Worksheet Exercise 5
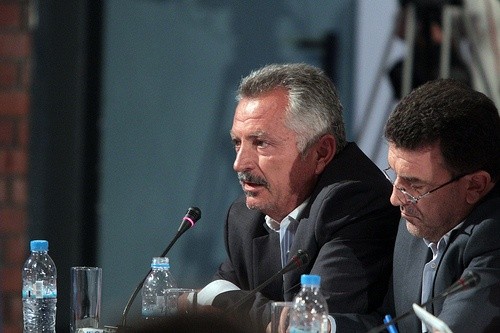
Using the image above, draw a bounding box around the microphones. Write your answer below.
[211,248,313,324]
[368,271,481,333]
[121,207,201,324]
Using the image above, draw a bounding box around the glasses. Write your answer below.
[384,166,471,204]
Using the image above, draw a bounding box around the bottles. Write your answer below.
[21,239,56,333]
[286,274,329,333]
[140,257,179,326]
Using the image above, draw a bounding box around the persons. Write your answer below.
[385,78,500,333]
[178,62,402,333]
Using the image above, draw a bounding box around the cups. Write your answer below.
[270,302,336,333]
[163,287,198,318]
[70,266,102,333]
